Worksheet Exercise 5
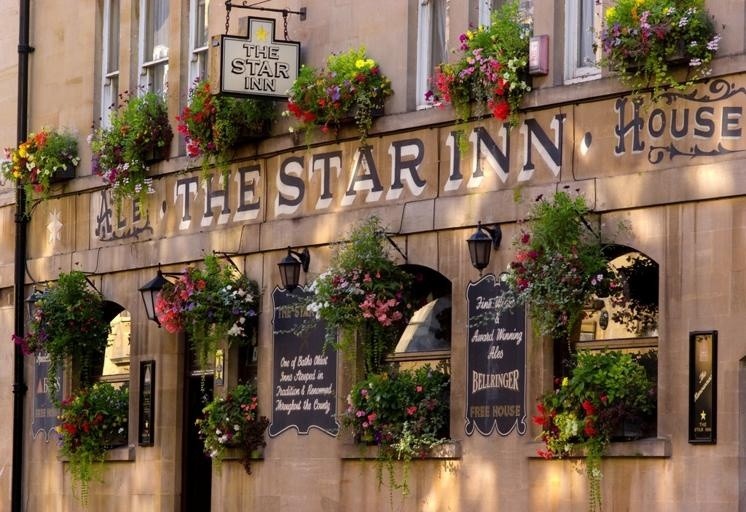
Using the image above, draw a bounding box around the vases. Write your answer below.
[469,69,532,100]
[142,140,165,161]
[613,34,695,72]
[19,158,75,185]
[236,119,270,138]
[319,97,384,127]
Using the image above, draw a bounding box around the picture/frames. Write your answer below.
[688,330,718,445]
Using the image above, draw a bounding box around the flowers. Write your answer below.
[584,0,723,114]
[175,75,282,193]
[0,124,80,221]
[281,42,394,158]
[86,82,174,218]
[425,0,534,161]
